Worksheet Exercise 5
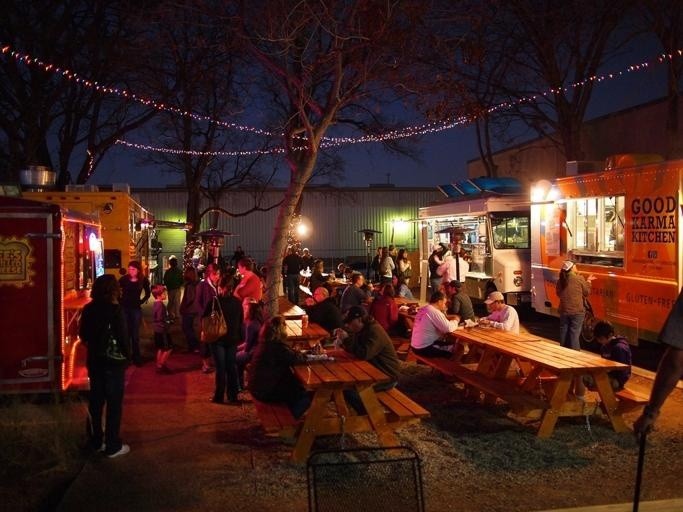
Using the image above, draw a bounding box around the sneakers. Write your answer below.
[207,395,225,404]
[154,363,176,376]
[105,444,131,459]
[200,362,217,374]
[91,438,107,454]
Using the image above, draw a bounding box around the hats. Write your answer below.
[483,290,505,305]
[560,259,575,272]
[445,279,462,288]
[340,305,368,324]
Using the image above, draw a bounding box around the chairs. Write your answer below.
[307,447,425,511]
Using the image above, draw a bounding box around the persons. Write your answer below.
[79,274,130,460]
[634,286,683,443]
[604,205,626,256]
[593,321,631,391]
[579,311,597,391]
[556,260,597,349]
[120,235,519,420]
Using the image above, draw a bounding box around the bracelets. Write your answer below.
[643,405,659,419]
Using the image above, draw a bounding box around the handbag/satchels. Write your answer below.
[103,335,133,366]
[199,309,229,344]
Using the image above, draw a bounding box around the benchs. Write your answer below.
[590,386,651,415]
[412,352,472,375]
[254,394,297,438]
[373,387,432,427]
[454,372,517,397]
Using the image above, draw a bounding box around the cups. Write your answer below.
[302,314,308,329]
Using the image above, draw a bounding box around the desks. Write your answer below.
[278,295,306,317]
[326,277,349,300]
[502,341,630,439]
[451,326,561,382]
[282,320,329,351]
[288,345,402,465]
[393,296,419,305]
[399,310,463,323]
